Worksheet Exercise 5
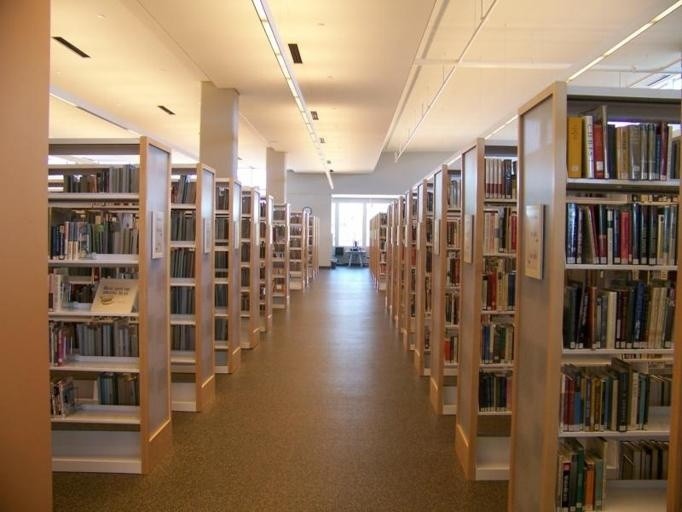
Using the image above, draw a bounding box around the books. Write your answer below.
[557,116,682,511]
[48,165,140,417]
[170,174,195,352]
[241,195,313,317]
[479,157,518,413]
[215,186,229,341]
[379,180,462,366]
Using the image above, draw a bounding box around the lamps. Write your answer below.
[251,0,334,190]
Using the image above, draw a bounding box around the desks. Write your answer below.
[345,249,367,267]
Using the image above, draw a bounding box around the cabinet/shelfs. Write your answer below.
[290,211,305,291]
[305,214,310,286]
[384,204,390,309]
[412,178,431,376]
[47,178,244,375]
[47,133,174,475]
[259,195,276,333]
[458,134,519,481]
[272,202,292,310]
[369,213,387,292]
[241,187,261,350]
[369,81,682,512]
[47,163,218,414]
[429,166,455,412]
[309,215,318,278]
[507,82,680,512]
[402,190,433,353]
[395,196,405,331]
[390,200,399,320]
